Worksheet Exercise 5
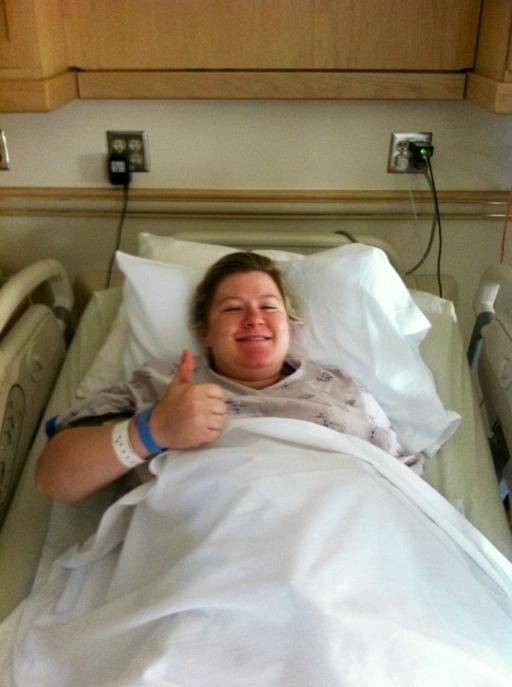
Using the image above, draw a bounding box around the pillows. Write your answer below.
[116,243,462,455]
[77,230,306,399]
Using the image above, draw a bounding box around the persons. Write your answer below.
[34,247,429,504]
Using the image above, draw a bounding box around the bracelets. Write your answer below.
[111,418,149,470]
[136,406,163,455]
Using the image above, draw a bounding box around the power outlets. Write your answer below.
[387,131,432,173]
[106,129,149,172]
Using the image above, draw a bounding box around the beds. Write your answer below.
[0,232,511,687]
